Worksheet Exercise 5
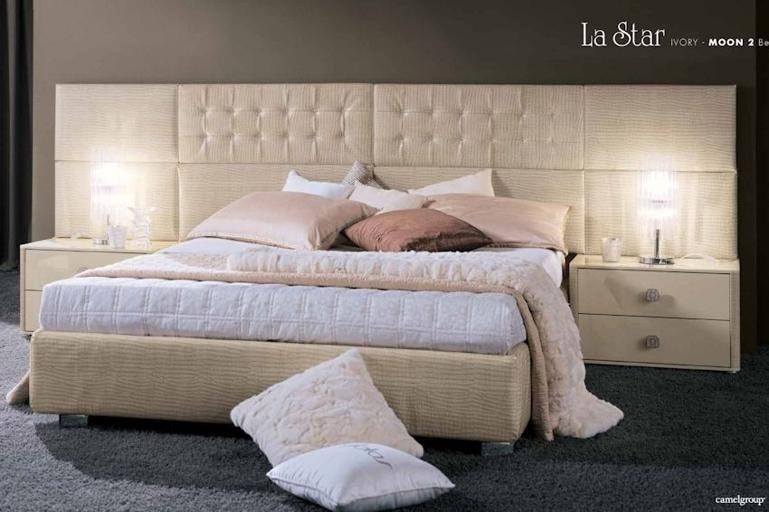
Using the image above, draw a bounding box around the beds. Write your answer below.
[10,81,738,458]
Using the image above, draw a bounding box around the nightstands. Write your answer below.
[17,232,169,336]
[565,253,740,377]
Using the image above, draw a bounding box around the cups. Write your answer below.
[107,222,125,249]
[601,236,623,261]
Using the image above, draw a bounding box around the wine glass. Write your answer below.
[125,203,157,249]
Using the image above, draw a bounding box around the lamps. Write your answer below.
[634,166,682,264]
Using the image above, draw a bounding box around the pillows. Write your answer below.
[266,438,457,512]
[227,345,422,465]
[345,181,427,211]
[342,209,491,252]
[284,169,354,199]
[408,166,498,198]
[421,194,578,252]
[176,188,379,251]
